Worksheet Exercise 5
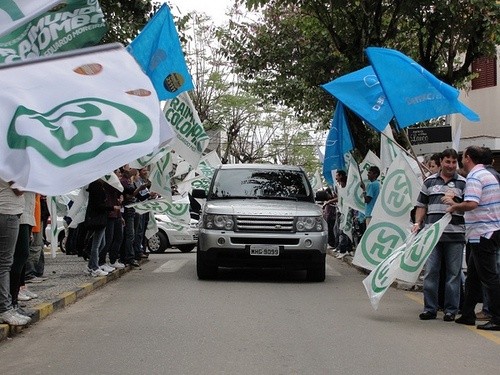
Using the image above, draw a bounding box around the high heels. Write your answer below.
[0,308,32,326]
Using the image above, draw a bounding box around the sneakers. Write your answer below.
[17,292,30,301]
[92,268,109,276]
[100,264,114,272]
[110,261,125,269]
[20,286,38,299]
[328,244,353,259]
[83,265,93,274]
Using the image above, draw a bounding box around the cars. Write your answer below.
[44,208,198,254]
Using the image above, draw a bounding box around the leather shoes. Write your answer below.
[456,317,475,325]
[477,321,500,330]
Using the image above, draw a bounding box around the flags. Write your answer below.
[0,41,177,198]
[0,0,110,65]
[45,138,226,259]
[321,99,427,273]
[388,211,452,284]
[160,89,211,170]
[364,45,482,128]
[123,2,195,102]
[317,64,395,133]
[0,0,57,31]
[362,231,418,311]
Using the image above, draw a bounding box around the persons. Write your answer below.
[0,152,183,328]
[313,141,499,332]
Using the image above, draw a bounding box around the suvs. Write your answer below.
[191,162,333,282]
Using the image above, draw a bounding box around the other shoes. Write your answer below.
[25,277,44,283]
[443,312,455,321]
[14,306,34,317]
[419,310,437,320]
[130,261,140,267]
